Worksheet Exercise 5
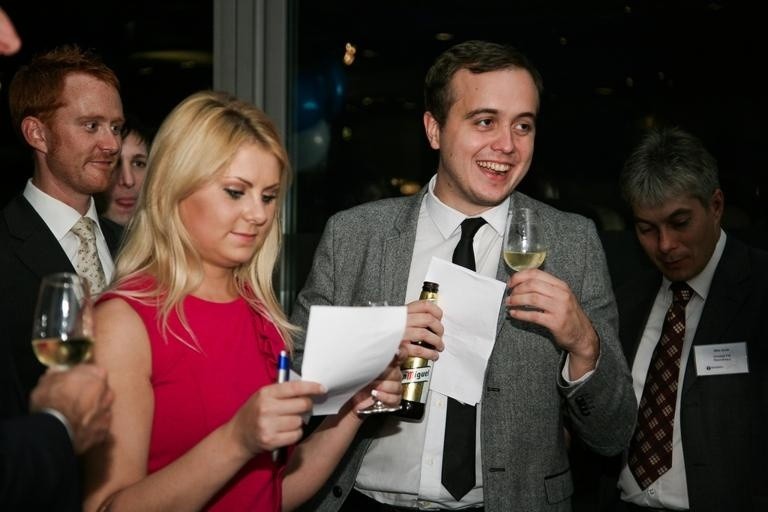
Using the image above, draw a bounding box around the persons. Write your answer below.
[100,120,152,228]
[81,92,409,512]
[572,128,768,512]
[287,39,637,511]
[0,43,126,512]
[0,7,113,512]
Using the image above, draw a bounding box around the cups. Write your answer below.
[503,208,549,274]
[31,273,95,370]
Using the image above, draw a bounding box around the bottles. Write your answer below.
[392,281,440,423]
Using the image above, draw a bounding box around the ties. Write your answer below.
[67,213,111,303]
[624,280,694,494]
[438,214,493,504]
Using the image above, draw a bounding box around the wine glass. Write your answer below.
[353,299,400,414]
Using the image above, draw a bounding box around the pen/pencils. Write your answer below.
[272,351,288,462]
